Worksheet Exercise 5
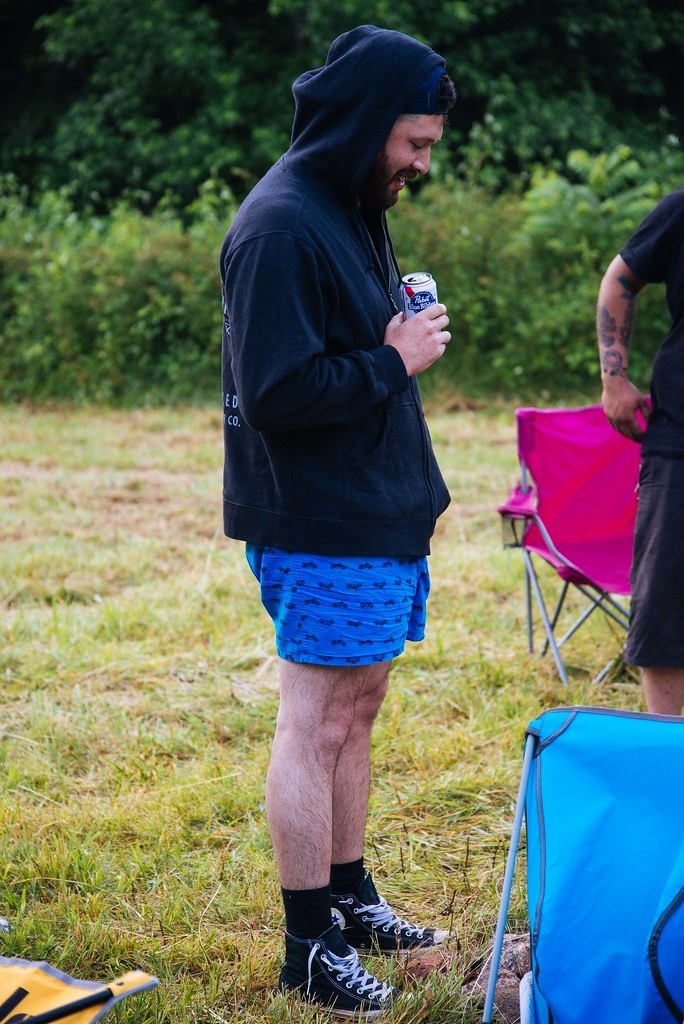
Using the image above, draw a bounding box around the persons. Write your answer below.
[222,31,465,1015]
[595,185,683,716]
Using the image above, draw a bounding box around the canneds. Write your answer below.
[399,272,438,322]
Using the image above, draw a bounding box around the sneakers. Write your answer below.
[329,873,453,955]
[277,922,427,1019]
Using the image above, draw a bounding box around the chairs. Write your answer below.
[480,705,684,1024]
[497,396,650,686]
